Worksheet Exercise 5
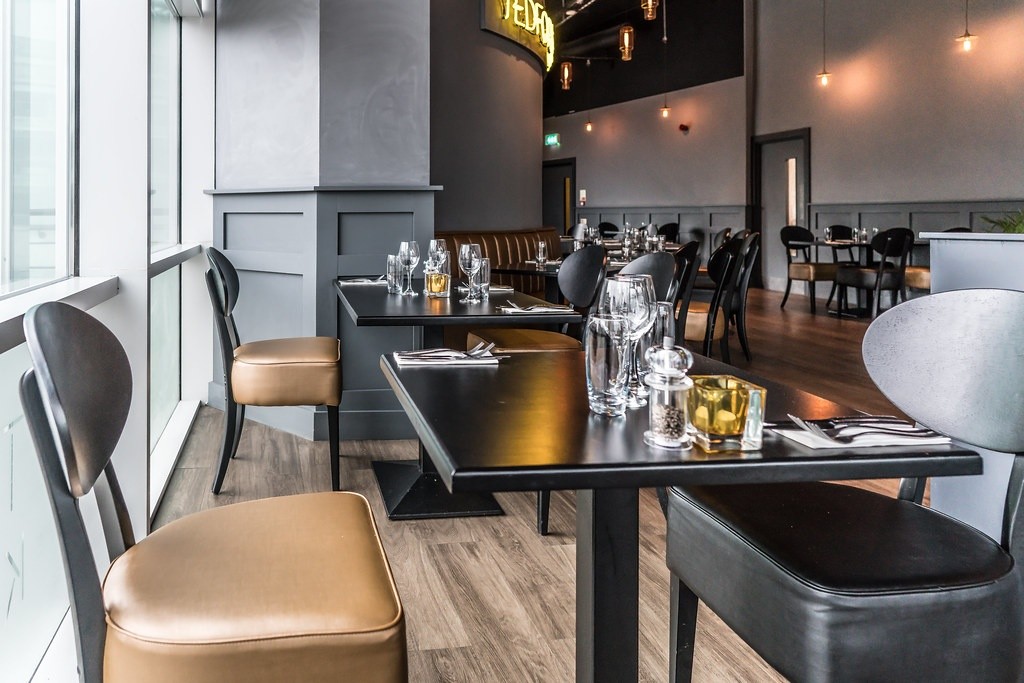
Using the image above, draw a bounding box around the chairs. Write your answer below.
[546,221,915,364]
[202,247,343,492]
[665,290,1024,683]
[17,302,409,683]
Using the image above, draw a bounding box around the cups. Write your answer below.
[425,273,450,297]
[631,302,676,396]
[472,258,489,302]
[585,313,630,417]
[852,227,879,244]
[535,241,547,268]
[574,222,668,262]
[428,239,447,264]
[386,254,403,294]
[684,375,767,454]
[824,228,832,242]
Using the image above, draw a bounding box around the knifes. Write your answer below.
[762,415,912,430]
[401,355,512,360]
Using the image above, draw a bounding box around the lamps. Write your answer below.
[640,0,659,20]
[620,22,635,61]
[560,62,572,90]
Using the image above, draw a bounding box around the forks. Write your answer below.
[395,342,496,358]
[787,414,948,445]
[507,299,569,311]
[338,274,385,282]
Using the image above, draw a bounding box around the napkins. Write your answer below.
[764,421,953,451]
[500,305,573,313]
[457,285,514,292]
[392,350,500,365]
[340,279,388,287]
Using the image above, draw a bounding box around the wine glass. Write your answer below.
[597,271,660,409]
[398,241,420,296]
[459,244,482,304]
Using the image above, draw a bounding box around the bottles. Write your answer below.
[644,335,695,450]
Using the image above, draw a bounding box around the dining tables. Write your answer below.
[380,342,982,683]
[790,235,932,318]
[487,260,631,330]
[332,269,584,520]
